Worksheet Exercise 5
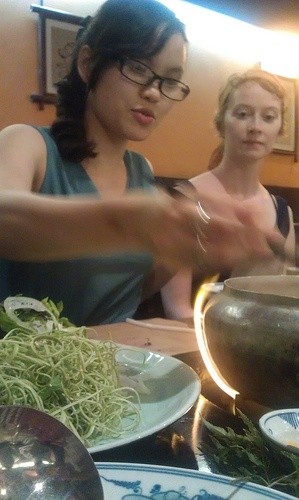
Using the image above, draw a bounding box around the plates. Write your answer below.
[0,338,201,470]
[258,408,299,453]
[93,462,299,500]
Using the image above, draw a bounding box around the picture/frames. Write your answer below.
[30,0,86,110]
[257,75,299,163]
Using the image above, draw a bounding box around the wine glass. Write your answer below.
[194,283,239,400]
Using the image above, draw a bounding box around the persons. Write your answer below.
[134,67,295,321]
[1,1,285,330]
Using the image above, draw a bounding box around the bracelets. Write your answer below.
[103,194,130,255]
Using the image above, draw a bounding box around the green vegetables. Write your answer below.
[0,295,143,447]
[197,407,299,488]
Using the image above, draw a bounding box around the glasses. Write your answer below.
[111,50,191,103]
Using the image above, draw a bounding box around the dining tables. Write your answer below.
[75,318,199,470]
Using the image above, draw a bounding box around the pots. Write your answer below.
[204,275,299,409]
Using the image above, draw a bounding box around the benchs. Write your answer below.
[133,176,299,320]
